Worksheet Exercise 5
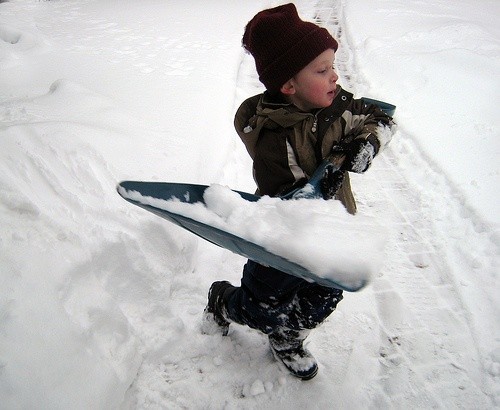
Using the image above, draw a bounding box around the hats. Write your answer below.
[240,3,338,93]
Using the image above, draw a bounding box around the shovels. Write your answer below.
[116,97,408,292]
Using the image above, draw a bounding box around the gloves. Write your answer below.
[321,169,346,201]
[331,132,380,174]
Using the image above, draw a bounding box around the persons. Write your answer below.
[201,3,397,382]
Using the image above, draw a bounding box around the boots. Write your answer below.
[269,336,319,381]
[199,281,235,337]
